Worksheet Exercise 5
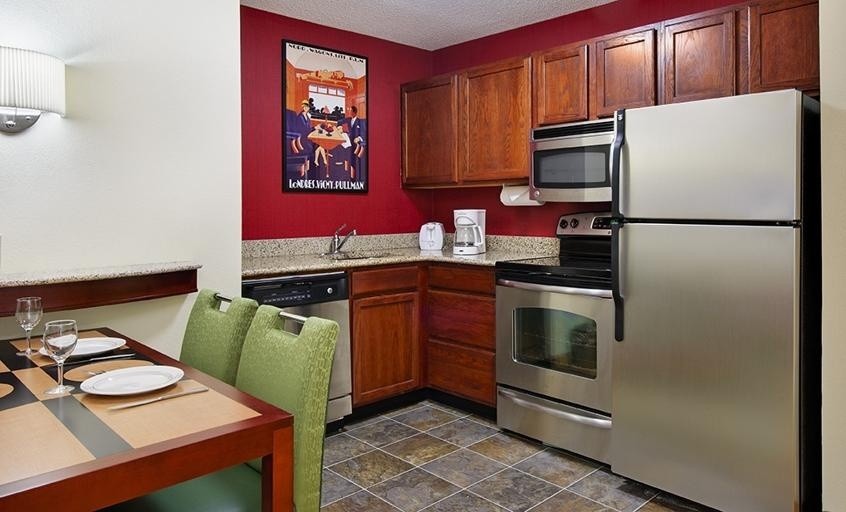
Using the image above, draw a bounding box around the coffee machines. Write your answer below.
[452,209,488,255]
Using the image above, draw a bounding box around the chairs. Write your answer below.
[179,289,260,386]
[137,306,340,511]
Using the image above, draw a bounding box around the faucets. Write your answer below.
[324,222,358,253]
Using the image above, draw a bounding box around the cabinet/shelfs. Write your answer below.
[398,56,531,190]
[534,1,819,123]
[425,262,496,420]
[350,260,421,419]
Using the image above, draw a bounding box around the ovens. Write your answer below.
[491,268,615,470]
[242,270,352,427]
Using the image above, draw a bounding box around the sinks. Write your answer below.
[327,247,397,265]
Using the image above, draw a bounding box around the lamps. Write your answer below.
[0,45,65,133]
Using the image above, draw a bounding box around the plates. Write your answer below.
[39,338,127,358]
[80,365,185,399]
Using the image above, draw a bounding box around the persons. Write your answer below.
[295,99,328,167]
[338,106,361,183]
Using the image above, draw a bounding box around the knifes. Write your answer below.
[52,354,137,369]
[108,387,210,412]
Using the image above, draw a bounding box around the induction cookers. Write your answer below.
[496,238,614,286]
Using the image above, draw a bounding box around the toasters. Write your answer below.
[418,221,445,251]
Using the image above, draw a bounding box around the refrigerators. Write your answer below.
[611,85,821,512]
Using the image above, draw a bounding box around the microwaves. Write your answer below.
[529,117,614,203]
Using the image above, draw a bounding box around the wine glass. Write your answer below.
[43,320,78,395]
[16,297,44,356]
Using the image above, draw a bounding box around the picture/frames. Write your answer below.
[281,40,370,195]
